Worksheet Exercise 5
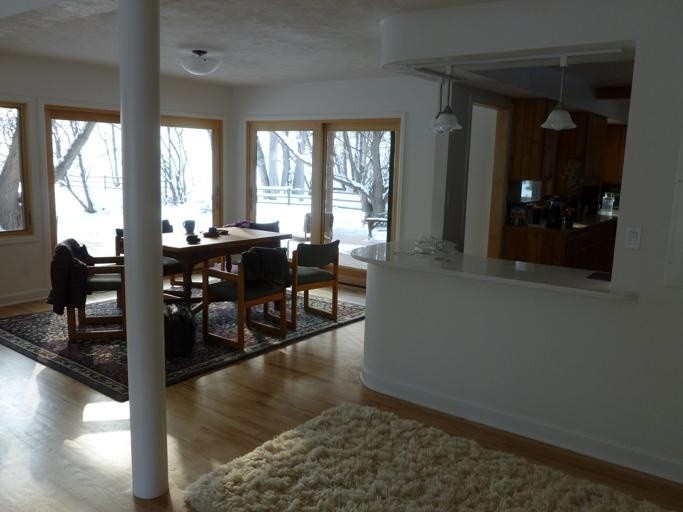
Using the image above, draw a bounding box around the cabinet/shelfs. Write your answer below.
[510,98,628,199]
[503,219,617,266]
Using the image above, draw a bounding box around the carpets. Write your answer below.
[0,278,366,403]
[184,402,669,512]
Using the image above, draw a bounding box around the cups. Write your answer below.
[184,220,195,235]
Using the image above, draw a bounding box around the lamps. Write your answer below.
[179,51,222,77]
[541,56,577,131]
[431,76,453,137]
[438,66,462,130]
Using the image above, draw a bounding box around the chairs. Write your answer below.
[162,220,186,287]
[248,221,280,249]
[56,239,127,342]
[202,248,287,350]
[116,229,124,308]
[264,240,340,329]
[287,213,334,259]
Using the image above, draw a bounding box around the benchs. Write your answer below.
[365,218,388,238]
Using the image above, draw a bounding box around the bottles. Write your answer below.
[602,192,614,216]
